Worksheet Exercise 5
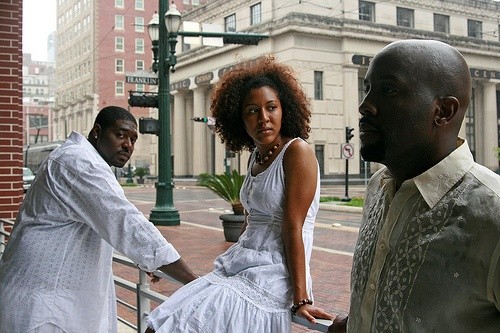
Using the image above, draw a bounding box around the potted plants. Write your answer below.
[192,168,249,242]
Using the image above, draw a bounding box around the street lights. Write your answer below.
[146,0,181,227]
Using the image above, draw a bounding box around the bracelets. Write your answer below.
[291,298,313,314]
[197,275,200,278]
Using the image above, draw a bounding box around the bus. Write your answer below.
[25,139,67,176]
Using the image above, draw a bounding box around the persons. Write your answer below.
[146,65,334,333]
[327,40,500,333]
[0,106,198,333]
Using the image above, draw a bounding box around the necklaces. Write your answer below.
[256,137,286,165]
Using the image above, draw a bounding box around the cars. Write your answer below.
[23,167,35,192]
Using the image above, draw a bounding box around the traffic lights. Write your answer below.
[191,116,208,123]
[345,126,355,143]
